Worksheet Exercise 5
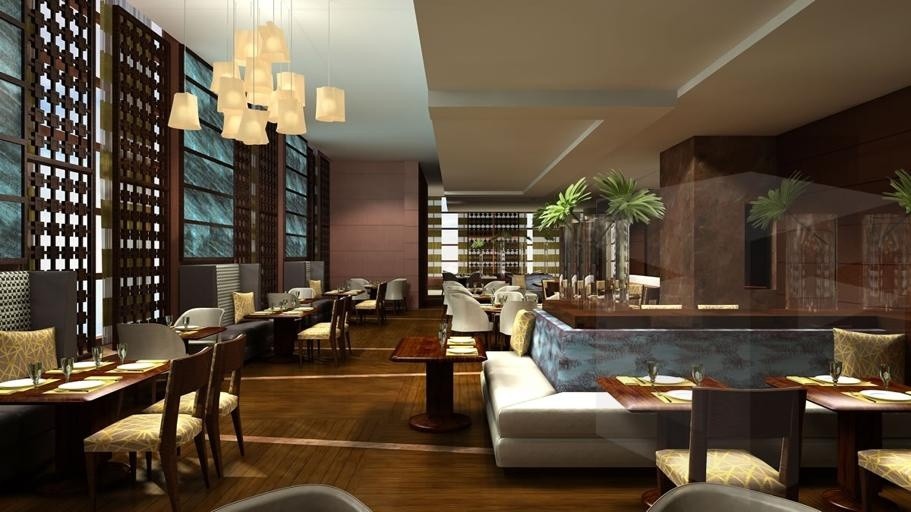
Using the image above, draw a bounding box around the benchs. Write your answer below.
[480,347,911,471]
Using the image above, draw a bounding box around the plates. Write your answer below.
[861,389,911,403]
[642,375,685,384]
[0,362,155,390]
[666,388,702,401]
[448,337,478,354]
[175,325,199,330]
[816,374,861,385]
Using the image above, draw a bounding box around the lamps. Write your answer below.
[166,0,347,146]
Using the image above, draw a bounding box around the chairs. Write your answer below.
[655,386,805,498]
[440,265,539,350]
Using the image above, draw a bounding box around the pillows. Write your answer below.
[510,310,537,356]
[831,326,908,386]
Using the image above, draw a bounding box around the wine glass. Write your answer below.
[469,261,519,268]
[644,363,662,387]
[436,321,450,352]
[90,344,102,371]
[691,363,707,389]
[116,342,127,365]
[164,314,189,333]
[268,291,301,313]
[60,356,74,380]
[879,363,891,391]
[829,361,842,390]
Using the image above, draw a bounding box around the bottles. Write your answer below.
[466,212,518,218]
[469,248,519,255]
